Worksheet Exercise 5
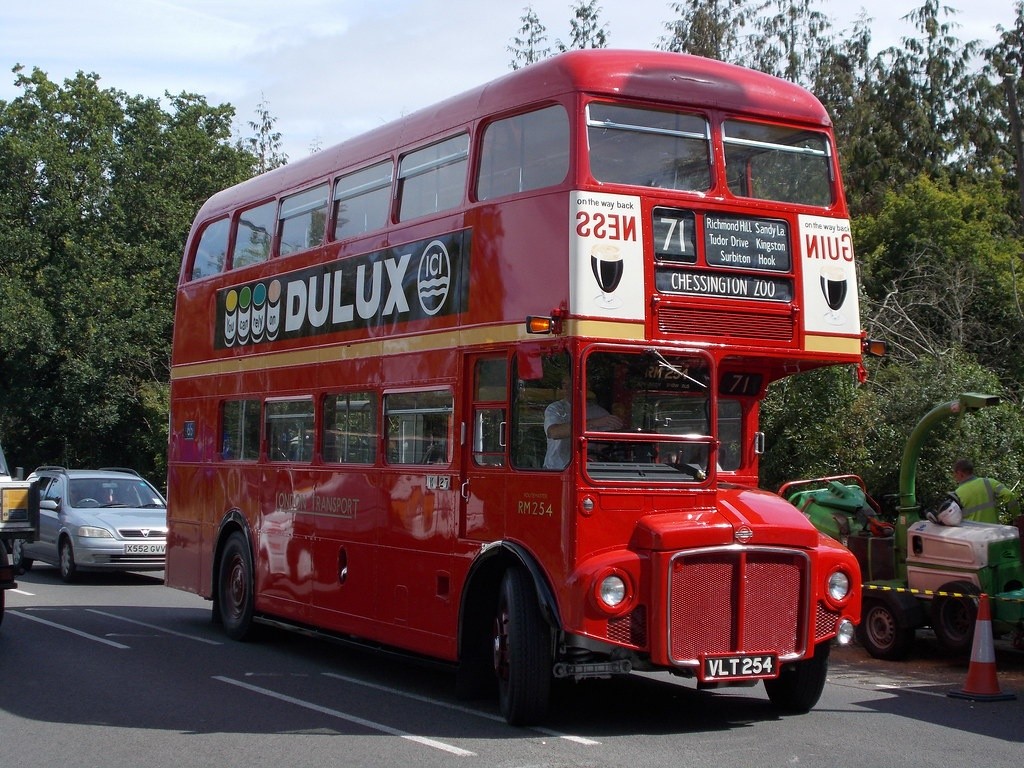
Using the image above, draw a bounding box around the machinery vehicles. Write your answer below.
[778,391,1024,665]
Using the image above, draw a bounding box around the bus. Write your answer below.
[163,49,888,731]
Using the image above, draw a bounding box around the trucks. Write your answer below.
[0,443,42,625]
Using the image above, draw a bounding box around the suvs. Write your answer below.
[9,465,170,583]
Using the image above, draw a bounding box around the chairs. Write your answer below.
[522,426,547,467]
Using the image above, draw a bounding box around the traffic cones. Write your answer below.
[946,593,1018,702]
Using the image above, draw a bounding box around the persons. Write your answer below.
[543,372,623,469]
[69,484,83,507]
[955,457,1014,524]
